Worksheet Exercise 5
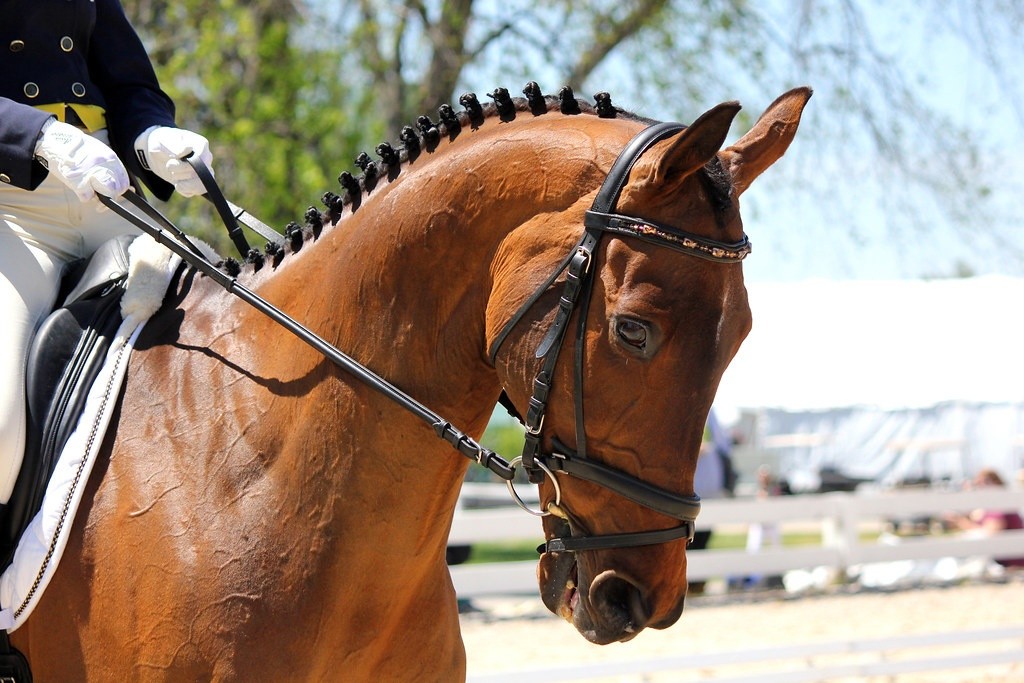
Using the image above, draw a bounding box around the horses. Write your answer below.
[0,80,813,683]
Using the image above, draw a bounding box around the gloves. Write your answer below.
[33,116,133,206]
[134,122,220,198]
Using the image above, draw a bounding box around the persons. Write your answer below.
[1,0,224,681]
[697,438,1023,592]
